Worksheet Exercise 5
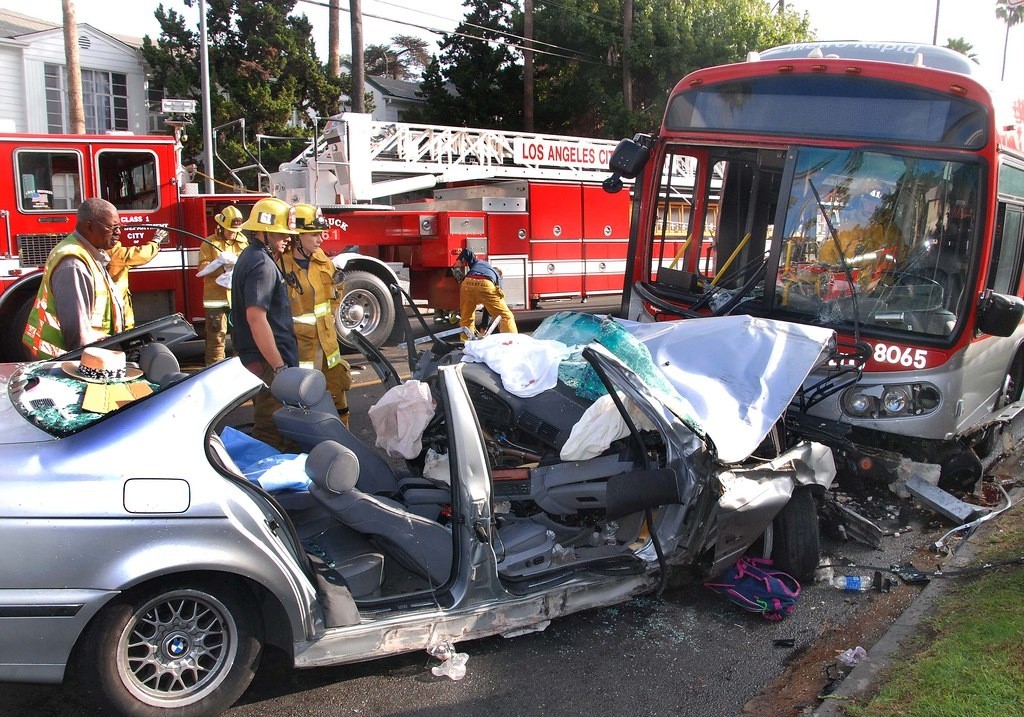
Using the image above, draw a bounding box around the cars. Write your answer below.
[0,312,839,717]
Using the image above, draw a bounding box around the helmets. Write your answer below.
[238,197,300,234]
[293,204,330,233]
[214,204,243,232]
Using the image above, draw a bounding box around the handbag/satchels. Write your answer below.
[704,552,800,623]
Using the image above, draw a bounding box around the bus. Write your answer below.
[600,37,1023,509]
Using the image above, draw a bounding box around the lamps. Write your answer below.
[161,99,196,194]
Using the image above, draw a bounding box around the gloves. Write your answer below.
[152,228,169,244]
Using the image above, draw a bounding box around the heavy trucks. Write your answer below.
[0,111,770,364]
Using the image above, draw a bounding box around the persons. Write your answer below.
[197,204,250,367]
[453,247,519,342]
[231,197,352,454]
[21,198,169,361]
[819,177,910,271]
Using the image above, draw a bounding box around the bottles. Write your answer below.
[875,312,904,323]
[829,575,872,591]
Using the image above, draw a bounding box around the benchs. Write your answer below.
[209,431,389,600]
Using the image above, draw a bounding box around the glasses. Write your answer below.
[91,220,121,233]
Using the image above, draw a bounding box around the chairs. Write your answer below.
[270,367,453,503]
[305,439,554,586]
